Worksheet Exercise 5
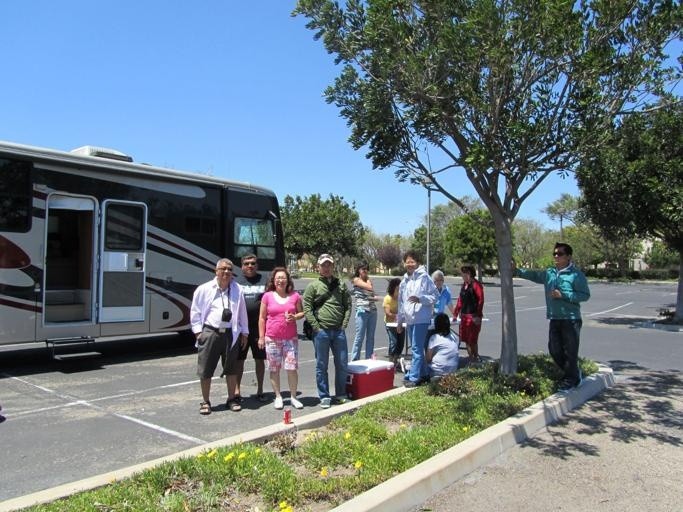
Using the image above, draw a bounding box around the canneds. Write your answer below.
[283,409,292,424]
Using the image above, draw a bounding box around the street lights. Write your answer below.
[556,206,565,243]
[419,174,435,277]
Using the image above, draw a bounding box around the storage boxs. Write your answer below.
[345,359,395,400]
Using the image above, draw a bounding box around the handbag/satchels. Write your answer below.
[304,320,311,340]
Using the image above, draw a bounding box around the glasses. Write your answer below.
[553,252,563,256]
[243,262,255,265]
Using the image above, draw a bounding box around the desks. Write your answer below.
[386,315,490,369]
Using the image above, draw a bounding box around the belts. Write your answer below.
[203,324,231,332]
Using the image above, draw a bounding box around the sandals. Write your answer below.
[200,401,210,414]
[227,397,245,411]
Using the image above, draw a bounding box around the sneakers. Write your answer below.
[319,397,352,408]
[275,398,303,409]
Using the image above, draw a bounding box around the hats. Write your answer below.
[317,253,333,264]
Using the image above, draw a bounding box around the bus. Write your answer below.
[0,141,288,363]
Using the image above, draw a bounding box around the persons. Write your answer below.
[424,313,459,380]
[510,242,590,392]
[397,250,438,388]
[257,268,305,410]
[431,268,455,317]
[347,257,380,360]
[233,251,268,405]
[302,254,351,409]
[188,257,249,415]
[452,265,484,359]
[382,279,401,372]
